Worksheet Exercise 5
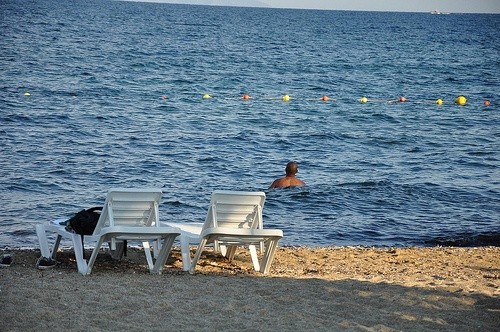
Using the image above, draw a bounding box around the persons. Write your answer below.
[269,162,305,188]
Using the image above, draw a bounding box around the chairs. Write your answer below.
[151,191,285,277]
[34,188,181,276]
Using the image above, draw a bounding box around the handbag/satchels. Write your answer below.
[60,207,104,235]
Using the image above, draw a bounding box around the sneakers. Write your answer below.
[35,257,58,270]
[0,254,12,267]
